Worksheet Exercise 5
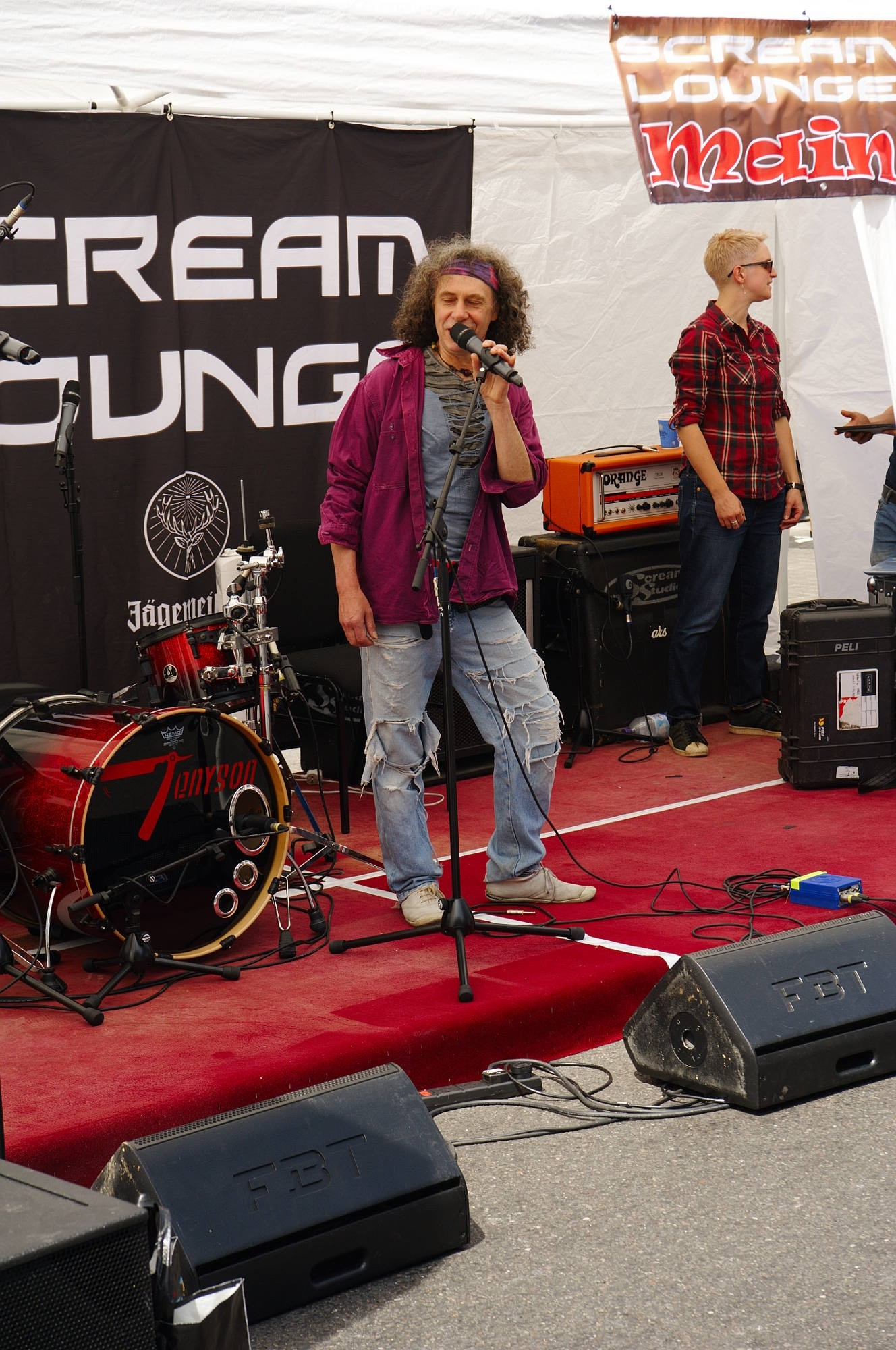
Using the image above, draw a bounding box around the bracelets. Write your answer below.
[787,483,804,492]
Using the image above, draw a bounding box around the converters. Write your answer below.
[789,872,863,910]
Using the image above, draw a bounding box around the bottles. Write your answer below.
[621,711,671,743]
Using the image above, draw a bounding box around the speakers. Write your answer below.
[619,908,896,1112]
[0,1063,470,1350]
[519,533,732,741]
[419,545,542,788]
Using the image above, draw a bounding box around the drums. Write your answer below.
[0,694,292,960]
[135,610,279,715]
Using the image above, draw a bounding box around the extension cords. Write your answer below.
[416,1072,542,1113]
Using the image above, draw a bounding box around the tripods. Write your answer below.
[542,552,667,768]
[77,352,584,1014]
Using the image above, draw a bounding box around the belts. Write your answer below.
[881,484,896,504]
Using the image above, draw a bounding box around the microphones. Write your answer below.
[53,380,81,468]
[449,322,524,388]
[0,331,42,365]
[617,575,634,629]
[241,814,290,834]
[0,192,34,243]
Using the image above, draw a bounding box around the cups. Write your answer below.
[657,418,679,449]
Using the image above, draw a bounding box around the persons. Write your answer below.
[832,406,896,567]
[318,243,596,927]
[667,228,804,757]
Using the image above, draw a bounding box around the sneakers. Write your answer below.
[668,718,708,757]
[484,859,597,904]
[729,700,782,738]
[401,881,446,927]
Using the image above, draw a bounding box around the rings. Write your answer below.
[731,521,738,525]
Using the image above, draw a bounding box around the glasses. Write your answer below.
[728,259,773,278]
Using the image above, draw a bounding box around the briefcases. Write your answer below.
[777,598,895,790]
